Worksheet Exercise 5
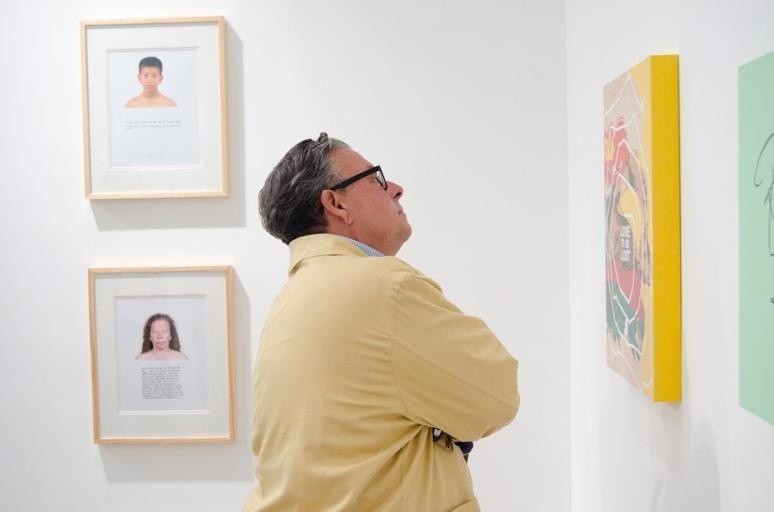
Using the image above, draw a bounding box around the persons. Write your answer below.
[122,55,178,109]
[133,313,189,362]
[241,129,522,512]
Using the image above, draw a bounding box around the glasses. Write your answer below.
[318,165,388,216]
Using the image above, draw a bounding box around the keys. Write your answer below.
[445,433,453,450]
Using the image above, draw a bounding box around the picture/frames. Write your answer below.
[79,14,232,202]
[86,264,239,447]
